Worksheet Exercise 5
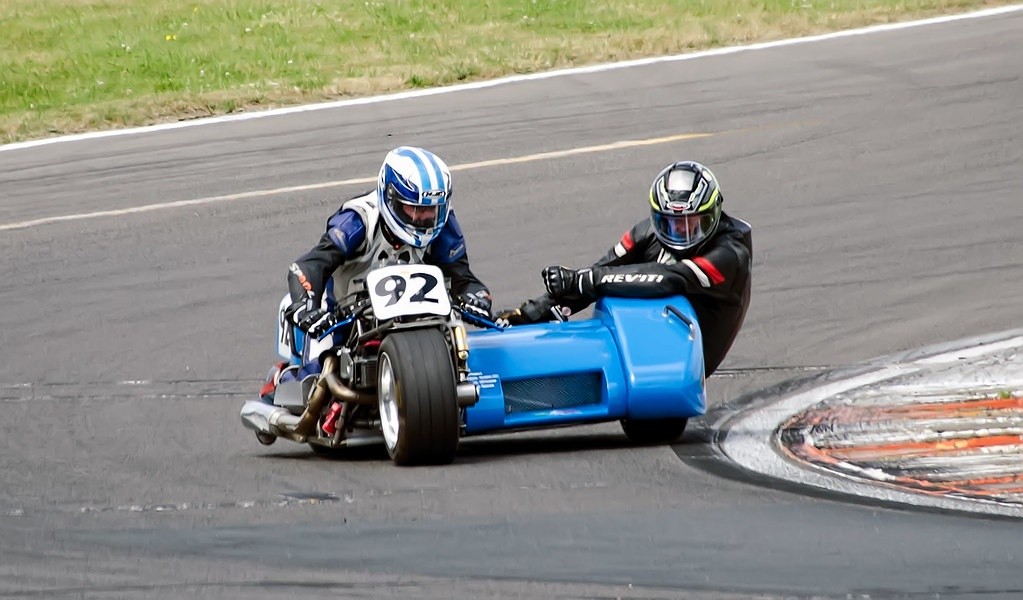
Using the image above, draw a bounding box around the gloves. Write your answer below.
[294,299,337,339]
[465,297,492,328]
[492,302,526,328]
[542,266,594,299]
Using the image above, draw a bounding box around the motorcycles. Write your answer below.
[239,257,479,467]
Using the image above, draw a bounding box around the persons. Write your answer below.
[496,161,753,379]
[255,145,492,445]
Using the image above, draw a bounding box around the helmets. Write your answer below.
[377,146,452,247]
[649,161,724,258]
